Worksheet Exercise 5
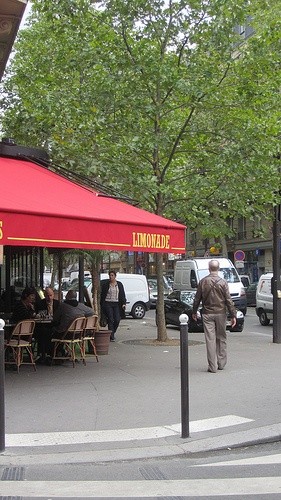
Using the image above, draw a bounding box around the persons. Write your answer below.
[100,271,126,342]
[9,287,96,366]
[191,260,236,372]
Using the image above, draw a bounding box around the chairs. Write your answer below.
[4,321,36,373]
[50,315,99,368]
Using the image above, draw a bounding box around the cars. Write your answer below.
[156,290,245,333]
[233,275,251,291]
[147,278,173,307]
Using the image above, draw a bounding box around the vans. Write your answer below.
[255,273,274,327]
[62,273,150,320]
[173,258,248,316]
[71,271,92,281]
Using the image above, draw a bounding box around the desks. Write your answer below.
[36,321,51,323]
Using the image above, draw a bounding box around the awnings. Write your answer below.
[0,157,186,254]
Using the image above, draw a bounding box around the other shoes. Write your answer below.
[110,336,115,341]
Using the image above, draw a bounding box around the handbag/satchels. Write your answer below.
[100,314,108,326]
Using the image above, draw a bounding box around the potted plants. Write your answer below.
[63,249,115,355]
